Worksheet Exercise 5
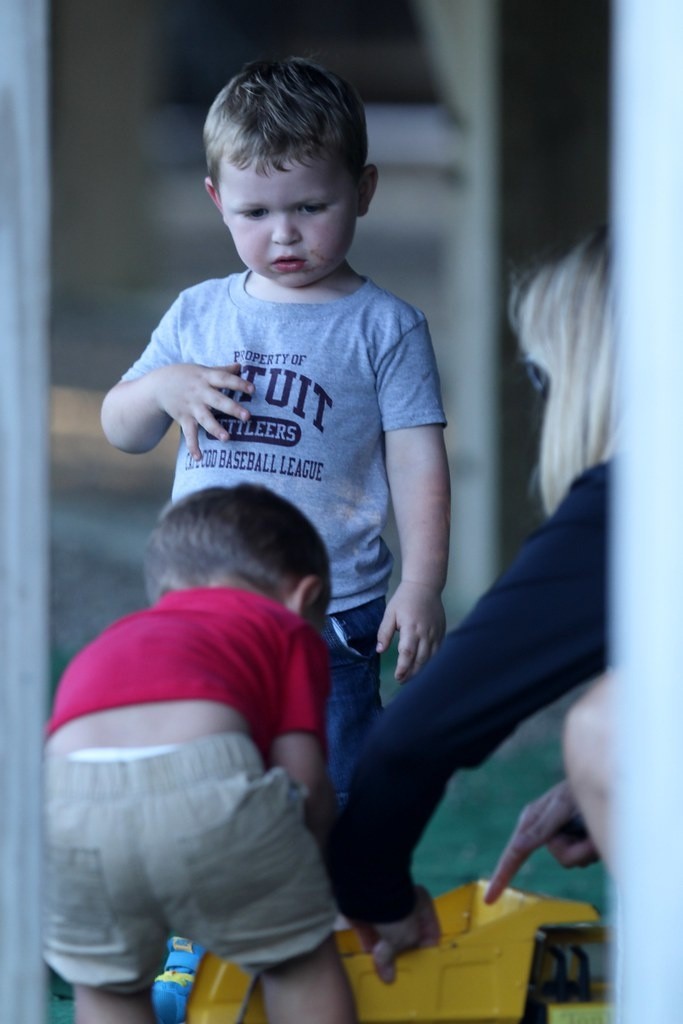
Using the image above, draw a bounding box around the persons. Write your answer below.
[98,59,450,1024]
[40,485,360,1024]
[332,229,621,985]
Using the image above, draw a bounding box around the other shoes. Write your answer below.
[150,935,206,1022]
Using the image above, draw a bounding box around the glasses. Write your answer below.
[525,356,550,399]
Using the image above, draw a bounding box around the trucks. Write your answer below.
[151,868,617,1024]
